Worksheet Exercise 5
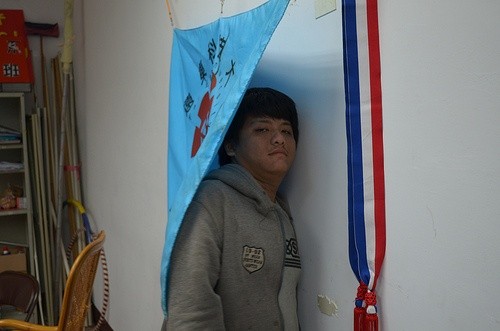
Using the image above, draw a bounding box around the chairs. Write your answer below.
[1,270,40,324]
[0,230,105,330]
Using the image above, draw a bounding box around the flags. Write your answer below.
[159,0,298,322]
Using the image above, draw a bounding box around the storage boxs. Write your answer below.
[1,8,34,91]
[0,248,28,275]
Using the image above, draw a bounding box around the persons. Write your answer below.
[161,85,305,330]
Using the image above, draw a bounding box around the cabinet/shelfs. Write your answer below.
[0,92,43,324]
